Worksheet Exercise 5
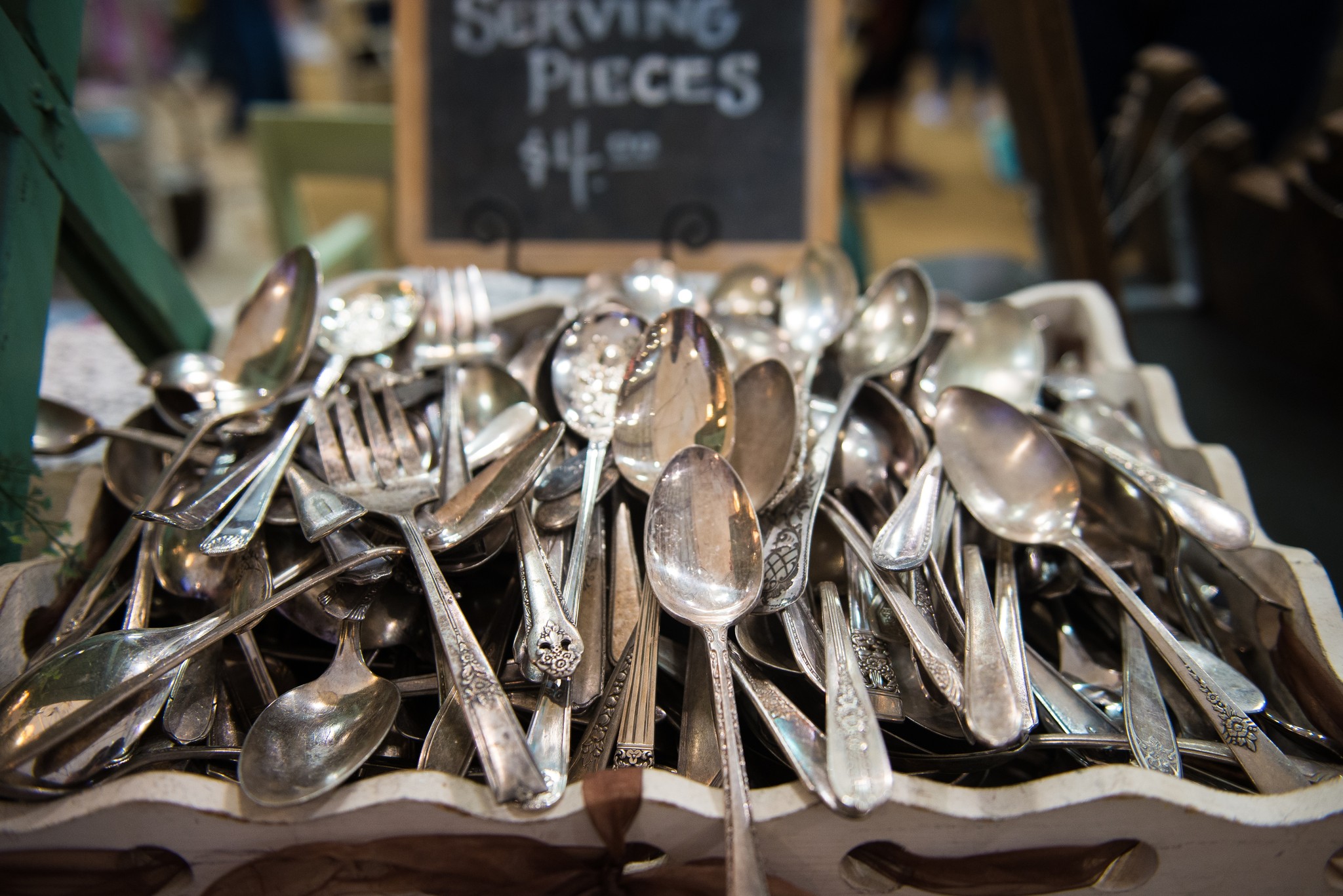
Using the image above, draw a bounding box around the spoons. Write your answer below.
[1,238,1342,896]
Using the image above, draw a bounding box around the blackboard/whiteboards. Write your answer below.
[386,0,846,285]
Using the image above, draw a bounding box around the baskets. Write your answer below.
[3,265,1343,896]
